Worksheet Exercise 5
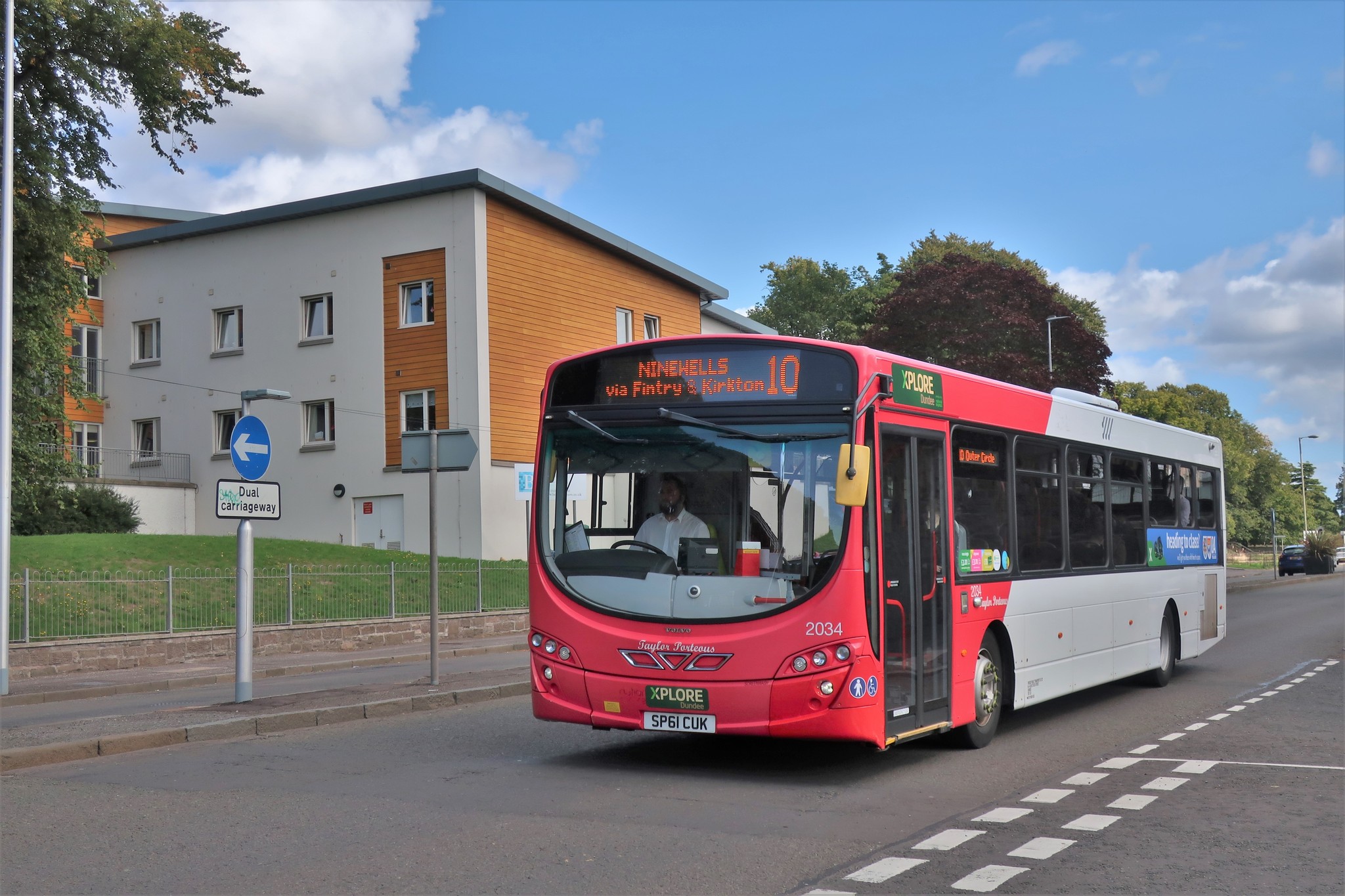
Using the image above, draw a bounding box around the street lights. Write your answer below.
[237,388,293,703]
[1299,435,1319,533]
[1046,315,1069,388]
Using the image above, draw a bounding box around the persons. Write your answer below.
[627,476,710,576]
[1179,475,1191,526]
[920,491,958,577]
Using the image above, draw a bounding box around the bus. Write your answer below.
[529,335,1226,754]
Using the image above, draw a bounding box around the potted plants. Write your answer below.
[1302,530,1341,575]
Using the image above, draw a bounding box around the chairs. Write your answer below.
[973,499,1215,573]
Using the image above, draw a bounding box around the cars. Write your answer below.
[1278,545,1306,576]
[1336,547,1345,565]
